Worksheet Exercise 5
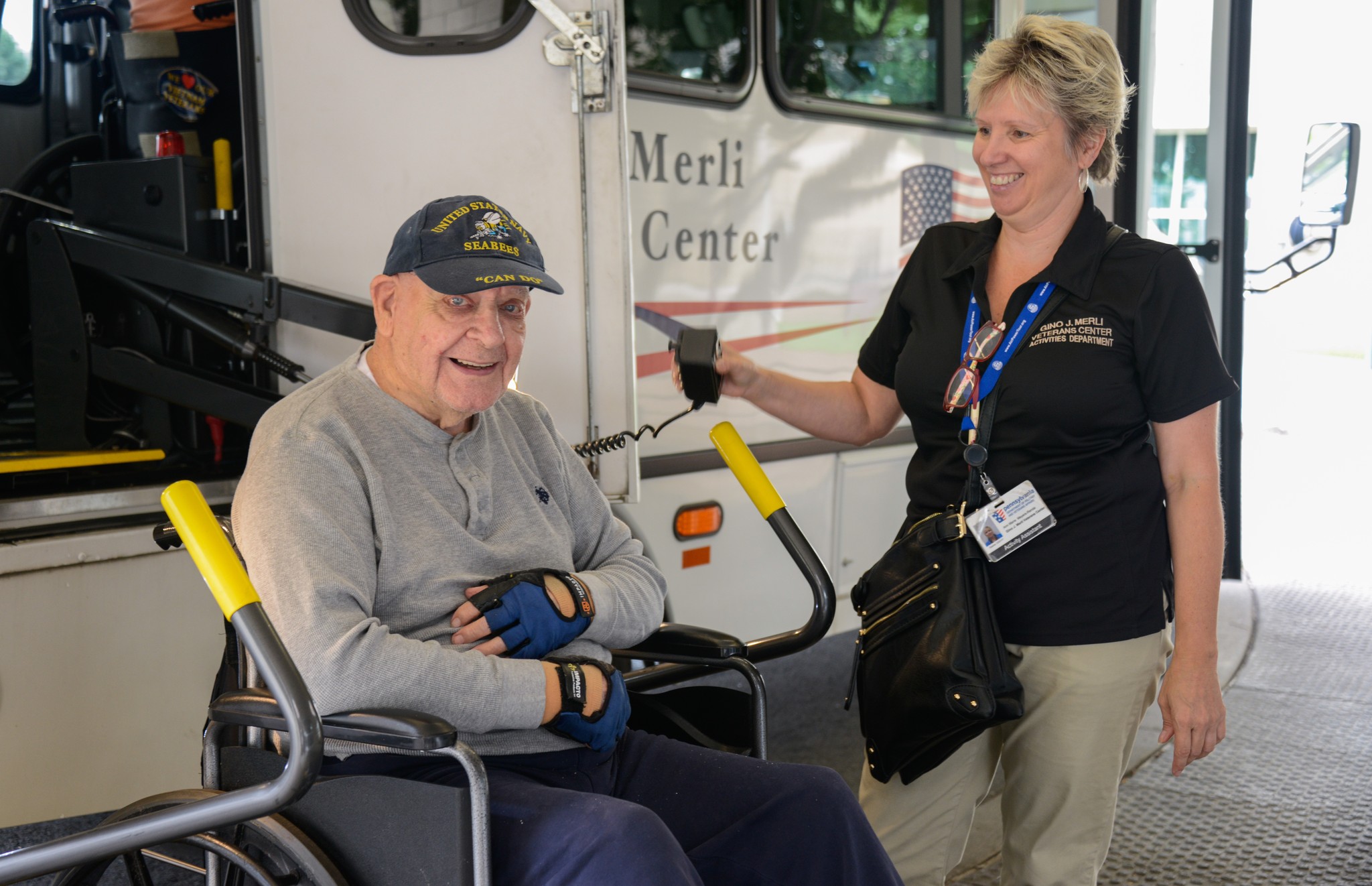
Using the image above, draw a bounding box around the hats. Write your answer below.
[382,195,566,299]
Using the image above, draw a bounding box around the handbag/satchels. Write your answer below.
[844,503,1025,787]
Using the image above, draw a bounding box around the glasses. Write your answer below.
[941,320,1007,413]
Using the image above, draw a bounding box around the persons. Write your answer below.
[669,14,1228,885]
[230,194,905,886]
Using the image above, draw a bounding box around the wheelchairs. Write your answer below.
[48,516,769,886]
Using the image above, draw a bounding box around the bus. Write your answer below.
[1,2,1360,854]
